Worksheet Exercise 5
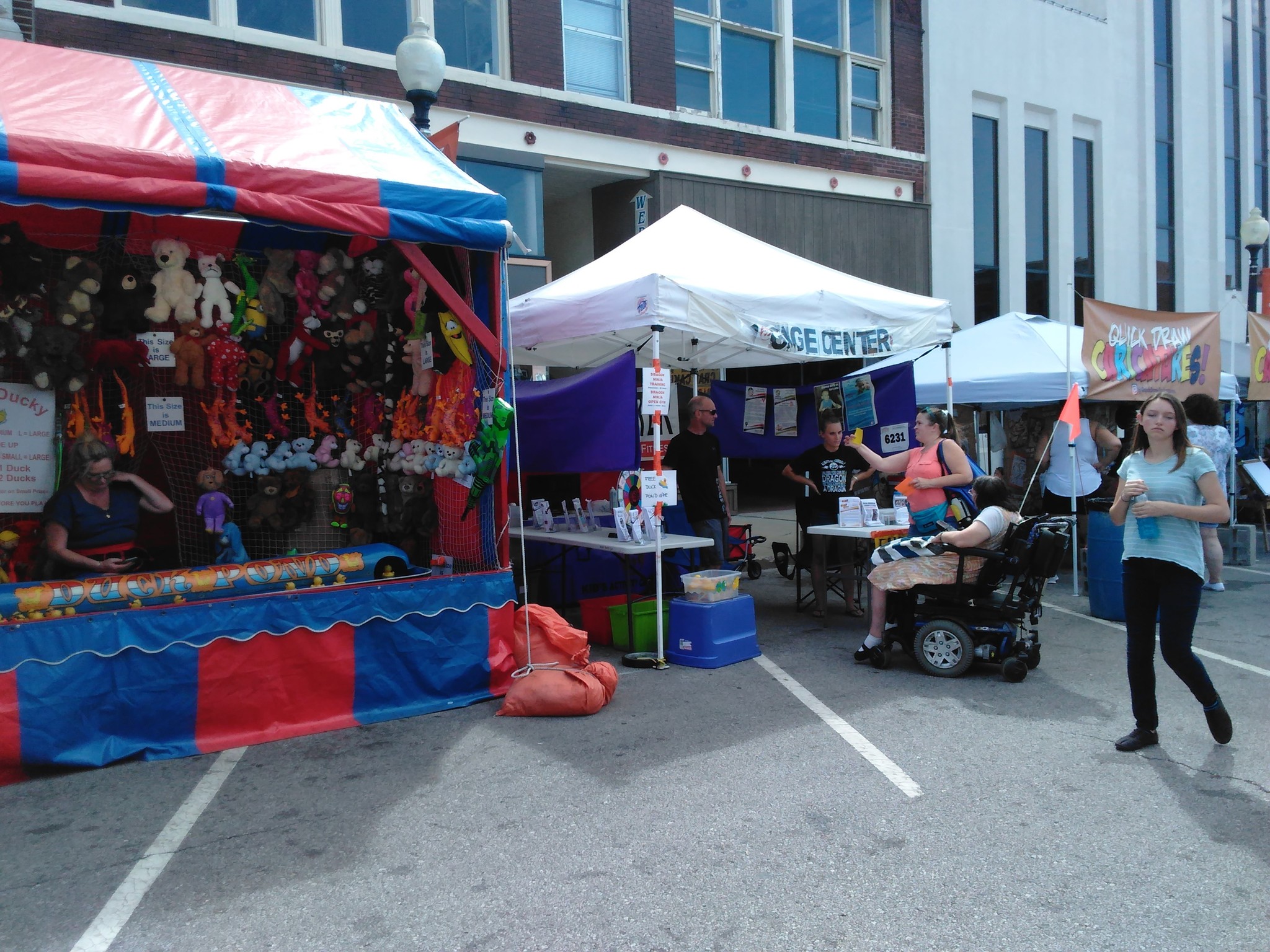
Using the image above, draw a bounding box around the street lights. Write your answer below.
[1240,206,1270,344]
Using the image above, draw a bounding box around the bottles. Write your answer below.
[1132,491,1159,540]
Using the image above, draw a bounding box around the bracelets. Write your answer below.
[939,530,948,544]
[1120,490,1132,502]
[97,559,103,573]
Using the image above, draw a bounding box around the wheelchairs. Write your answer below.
[867,510,1076,681]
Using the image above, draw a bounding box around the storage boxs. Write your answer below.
[680,569,742,603]
[579,594,656,645]
[665,594,761,668]
[606,600,671,654]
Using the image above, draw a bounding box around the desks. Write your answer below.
[508,525,714,670]
[807,523,910,631]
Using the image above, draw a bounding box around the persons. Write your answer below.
[818,390,841,415]
[842,405,976,545]
[855,378,870,396]
[1178,393,1236,591]
[1108,392,1236,750]
[781,411,879,618]
[41,433,174,583]
[849,475,1027,660]
[660,396,732,571]
[1034,406,1121,584]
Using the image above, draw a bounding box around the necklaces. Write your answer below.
[83,484,114,518]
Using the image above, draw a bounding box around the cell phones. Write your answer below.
[115,557,138,568]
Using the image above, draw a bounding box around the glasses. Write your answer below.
[86,468,116,481]
[968,489,978,496]
[694,409,717,415]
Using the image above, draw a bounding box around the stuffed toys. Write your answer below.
[0,218,500,582]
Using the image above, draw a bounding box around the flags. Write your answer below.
[1057,383,1082,440]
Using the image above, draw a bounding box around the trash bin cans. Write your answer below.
[1086,496,1165,622]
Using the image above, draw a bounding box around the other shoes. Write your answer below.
[854,643,893,661]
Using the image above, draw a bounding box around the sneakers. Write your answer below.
[1114,727,1159,750]
[1204,694,1234,744]
[1047,574,1059,584]
[1202,579,1225,592]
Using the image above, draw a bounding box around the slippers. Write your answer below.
[812,609,824,617]
[846,605,865,618]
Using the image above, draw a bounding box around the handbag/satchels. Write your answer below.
[938,439,988,518]
[906,501,949,533]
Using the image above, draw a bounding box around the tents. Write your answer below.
[507,202,958,672]
[911,310,1245,599]
